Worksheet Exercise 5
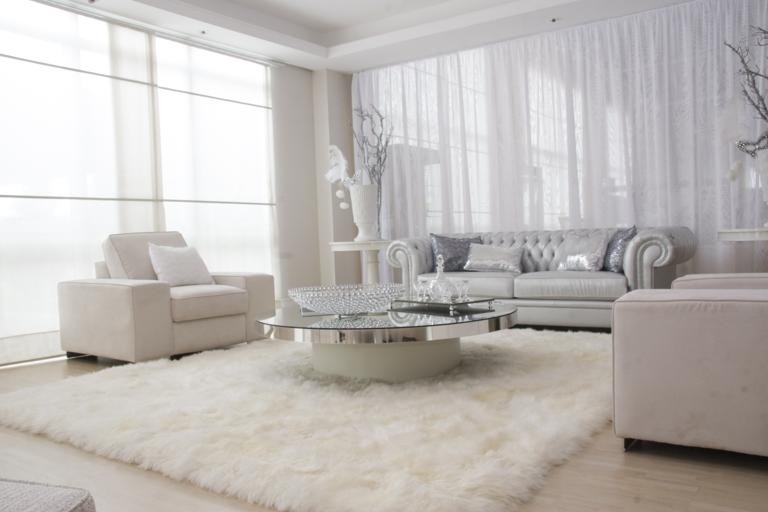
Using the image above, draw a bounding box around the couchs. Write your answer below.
[59,231,275,363]
[387,227,698,326]
[614,273,768,451]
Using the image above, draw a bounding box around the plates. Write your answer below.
[288,284,407,314]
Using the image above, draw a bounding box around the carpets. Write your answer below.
[0,326,612,511]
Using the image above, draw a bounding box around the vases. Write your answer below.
[349,183,380,243]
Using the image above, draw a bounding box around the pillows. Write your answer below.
[463,243,525,272]
[147,242,215,284]
[549,235,607,271]
[430,231,481,272]
[605,226,638,271]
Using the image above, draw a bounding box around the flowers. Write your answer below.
[349,107,392,180]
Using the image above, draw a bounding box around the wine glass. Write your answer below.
[453,279,463,301]
[462,280,471,300]
[420,282,428,302]
[412,281,421,301]
